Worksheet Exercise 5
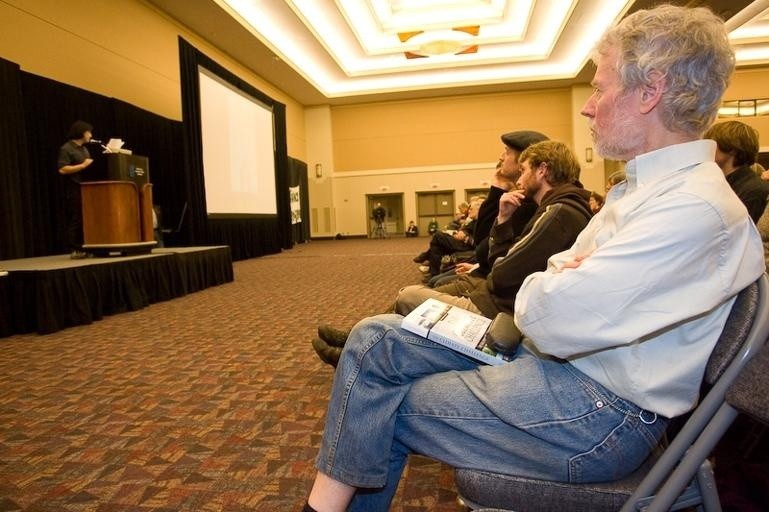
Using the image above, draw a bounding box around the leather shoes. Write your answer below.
[312,325,349,369]
[413,252,432,283]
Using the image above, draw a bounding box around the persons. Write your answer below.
[706,121,769,229]
[57,122,100,259]
[312,132,627,367]
[301,5,769,512]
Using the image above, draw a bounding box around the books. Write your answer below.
[102,136,132,154]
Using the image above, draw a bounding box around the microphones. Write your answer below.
[89,139,102,144]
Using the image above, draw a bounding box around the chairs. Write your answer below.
[454,270,768,512]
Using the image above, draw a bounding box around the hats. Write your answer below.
[501,130,550,151]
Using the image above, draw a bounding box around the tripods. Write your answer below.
[372,214,388,239]
